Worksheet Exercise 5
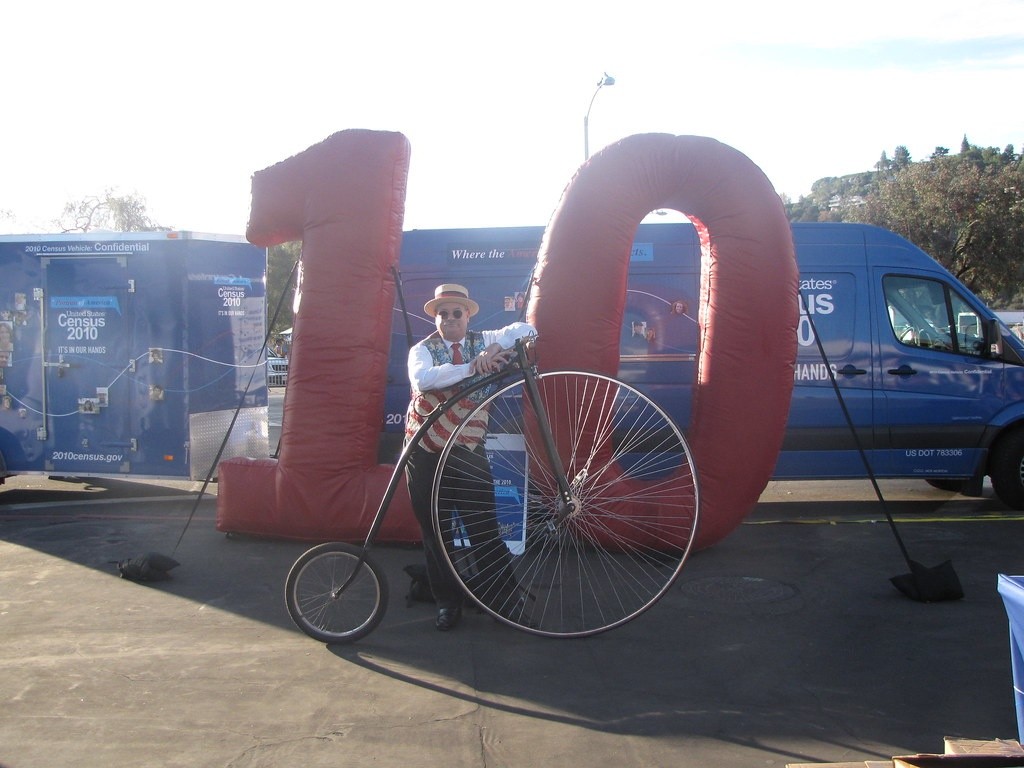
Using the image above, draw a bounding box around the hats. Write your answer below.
[423,284,479,317]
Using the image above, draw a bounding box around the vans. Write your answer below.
[379,224,1024,513]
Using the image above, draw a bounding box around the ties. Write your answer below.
[450,343,465,365]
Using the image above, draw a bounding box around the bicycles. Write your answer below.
[279,330,700,647]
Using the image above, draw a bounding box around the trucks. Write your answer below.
[2,228,269,505]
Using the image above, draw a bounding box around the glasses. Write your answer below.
[437,311,465,318]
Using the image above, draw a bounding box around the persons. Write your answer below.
[671,300,688,314]
[632,321,645,338]
[646,328,655,341]
[276,338,289,358]
[402,284,539,630]
[505,298,515,311]
[0,286,165,419]
[515,293,528,308]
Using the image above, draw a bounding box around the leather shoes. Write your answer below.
[493,608,542,628]
[434,606,463,630]
